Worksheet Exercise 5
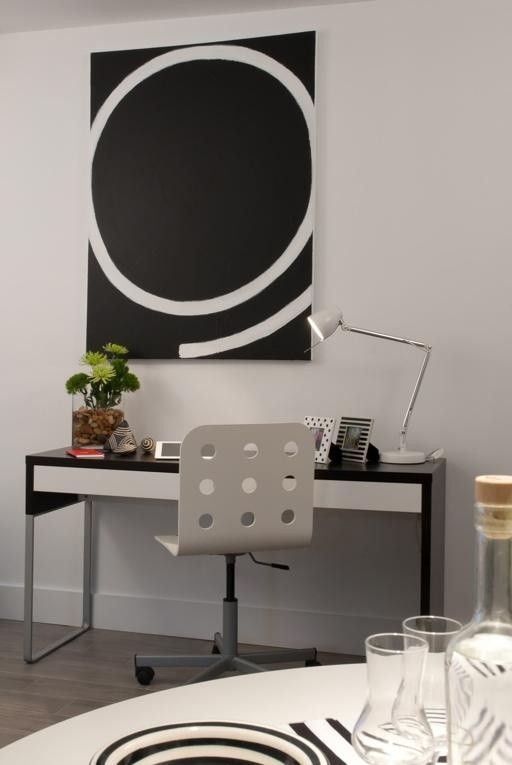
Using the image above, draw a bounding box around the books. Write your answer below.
[66,449,105,459]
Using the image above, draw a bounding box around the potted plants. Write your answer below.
[65,342,140,449]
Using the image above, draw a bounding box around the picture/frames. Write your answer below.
[302,416,342,464]
[337,416,380,463]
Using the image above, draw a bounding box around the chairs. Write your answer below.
[134,422,322,686]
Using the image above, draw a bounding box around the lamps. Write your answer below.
[306,308,432,464]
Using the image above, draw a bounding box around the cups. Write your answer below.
[392,617,463,745]
[349,635,433,765]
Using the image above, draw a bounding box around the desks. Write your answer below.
[22,445,447,664]
[0,652,511,765]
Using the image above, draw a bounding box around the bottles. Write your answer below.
[441,474,511,765]
